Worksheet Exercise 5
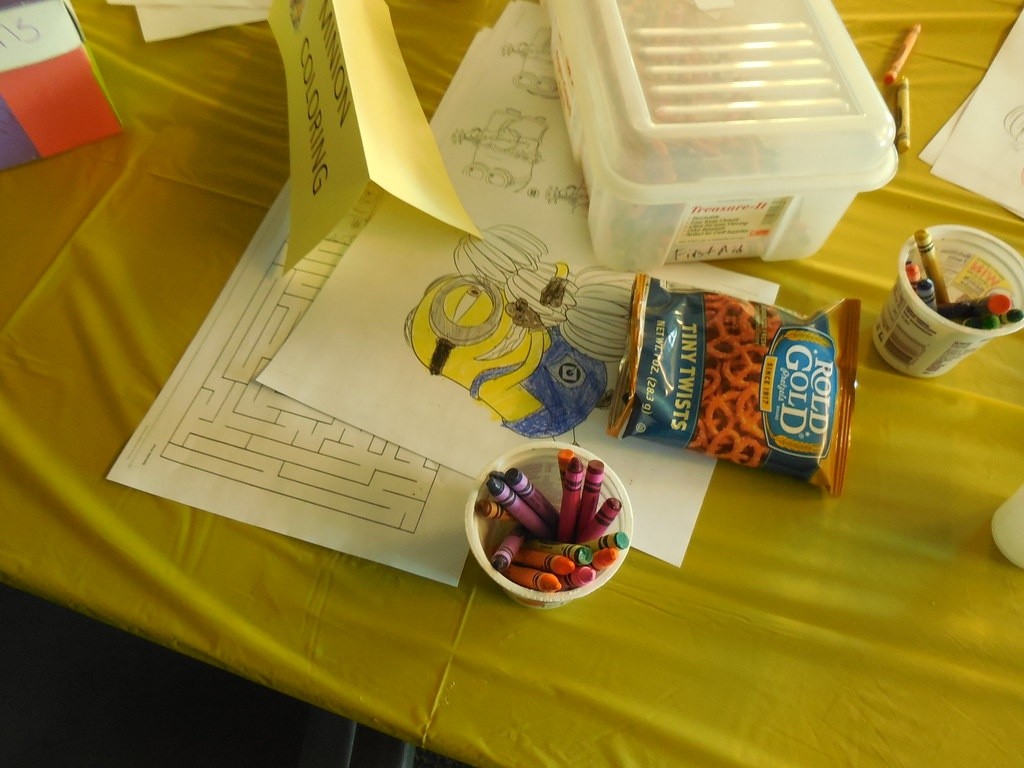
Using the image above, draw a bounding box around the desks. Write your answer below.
[0,0,1024,768]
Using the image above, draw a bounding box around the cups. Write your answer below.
[872,224,1024,379]
[465,440,634,609]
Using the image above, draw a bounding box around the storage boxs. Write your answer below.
[549,0,899,272]
[0,0,124,172]
